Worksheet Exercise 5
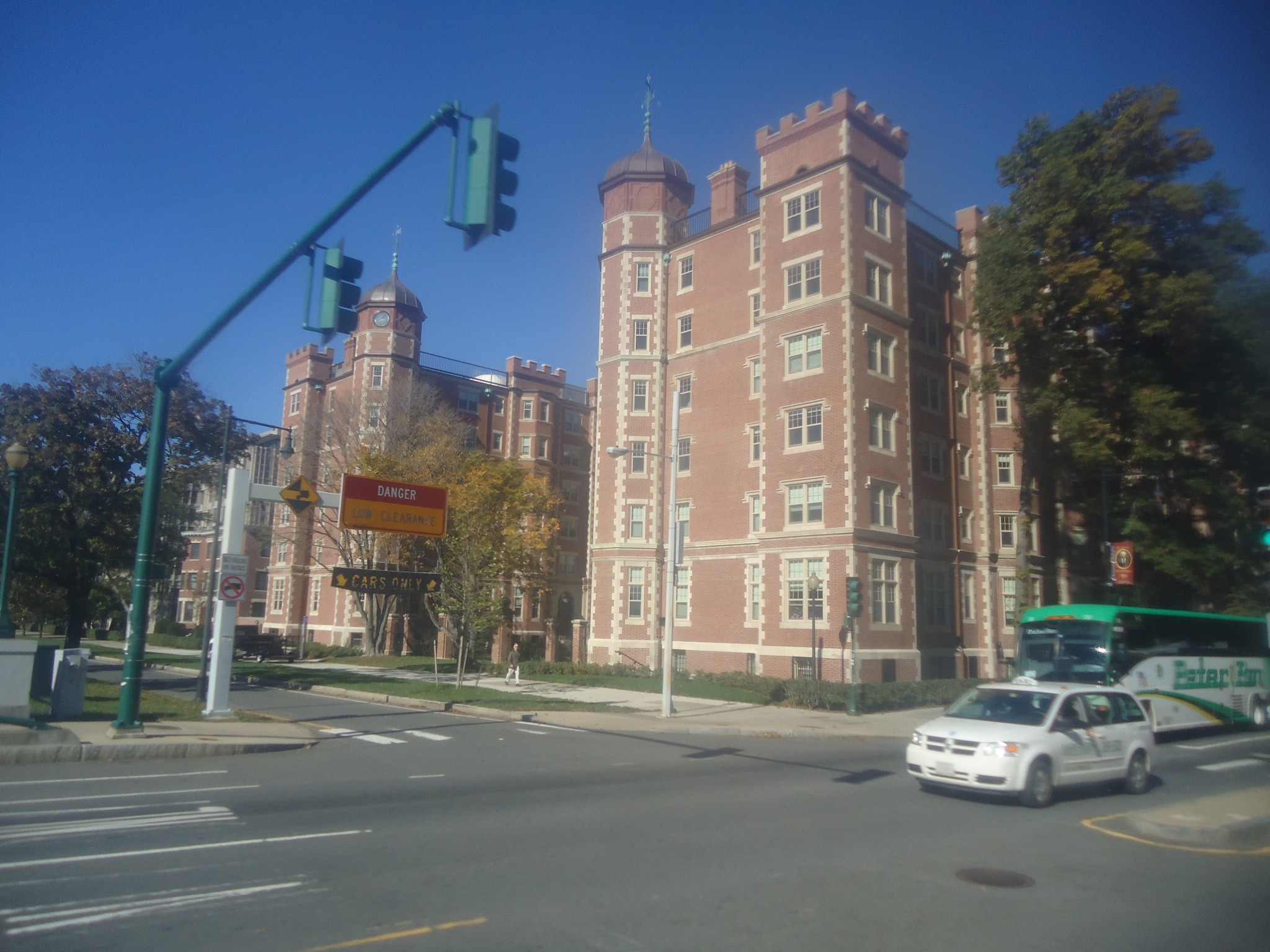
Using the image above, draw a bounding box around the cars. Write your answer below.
[906,675,1155,805]
[232,633,293,662]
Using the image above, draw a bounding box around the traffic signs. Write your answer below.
[332,566,441,595]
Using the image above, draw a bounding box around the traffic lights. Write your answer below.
[320,238,364,345]
[465,118,521,250]
[846,576,862,618]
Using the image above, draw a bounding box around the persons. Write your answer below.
[1057,697,1079,725]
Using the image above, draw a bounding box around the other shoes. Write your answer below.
[516,683,521,686]
[505,681,508,685]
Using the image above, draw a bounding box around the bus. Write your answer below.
[1004,606,1270,737]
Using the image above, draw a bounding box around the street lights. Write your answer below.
[196,404,295,703]
[807,571,821,707]
[606,391,681,716]
[0,442,29,637]
